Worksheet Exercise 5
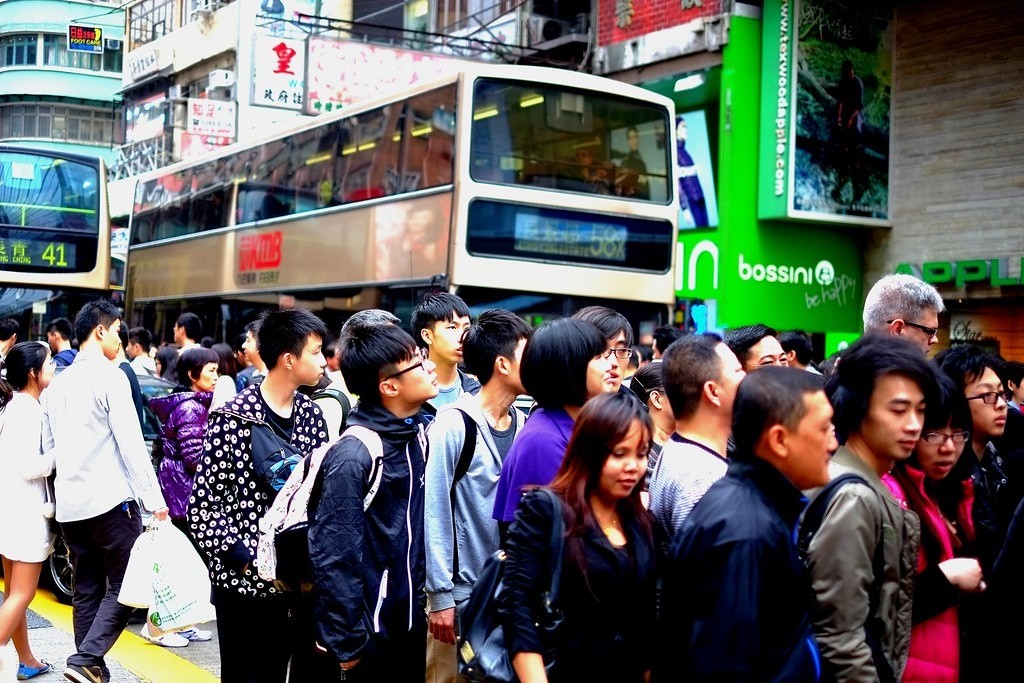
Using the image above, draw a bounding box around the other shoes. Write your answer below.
[16,660,50,679]
[173,627,212,640]
[140,624,189,646]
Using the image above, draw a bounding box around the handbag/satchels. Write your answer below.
[252,425,305,499]
[117,533,151,608]
[454,489,564,683]
[147,519,218,636]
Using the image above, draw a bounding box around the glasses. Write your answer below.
[887,320,938,339]
[921,431,971,445]
[605,347,633,359]
[966,391,1012,404]
[385,347,429,381]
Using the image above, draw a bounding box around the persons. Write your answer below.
[675,117,709,227]
[567,147,592,178]
[620,127,650,200]
[824,59,868,201]
[0,274,1024,683]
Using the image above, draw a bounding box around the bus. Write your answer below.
[122,64,679,353]
[0,142,110,352]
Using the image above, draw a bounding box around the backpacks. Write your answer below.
[258,425,383,581]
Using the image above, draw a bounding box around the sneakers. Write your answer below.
[64,662,109,683]
